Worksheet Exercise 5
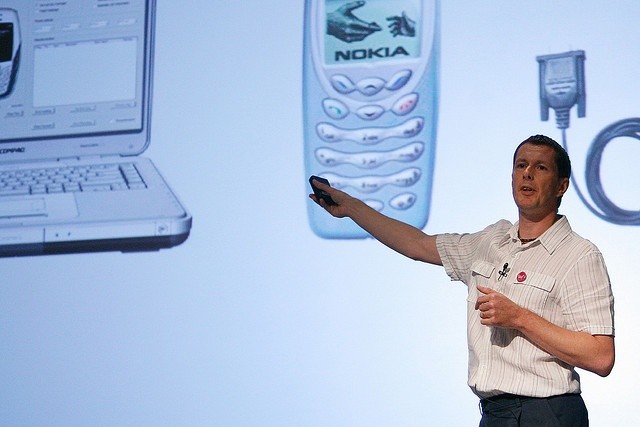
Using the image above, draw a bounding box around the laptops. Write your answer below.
[0,0,193,258]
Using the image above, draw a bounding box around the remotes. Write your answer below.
[309,175,335,206]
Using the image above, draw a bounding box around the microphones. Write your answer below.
[497,263,508,281]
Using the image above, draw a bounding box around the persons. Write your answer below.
[308,135,616,426]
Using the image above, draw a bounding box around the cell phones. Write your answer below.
[301,0,442,240]
[0,7,22,97]
